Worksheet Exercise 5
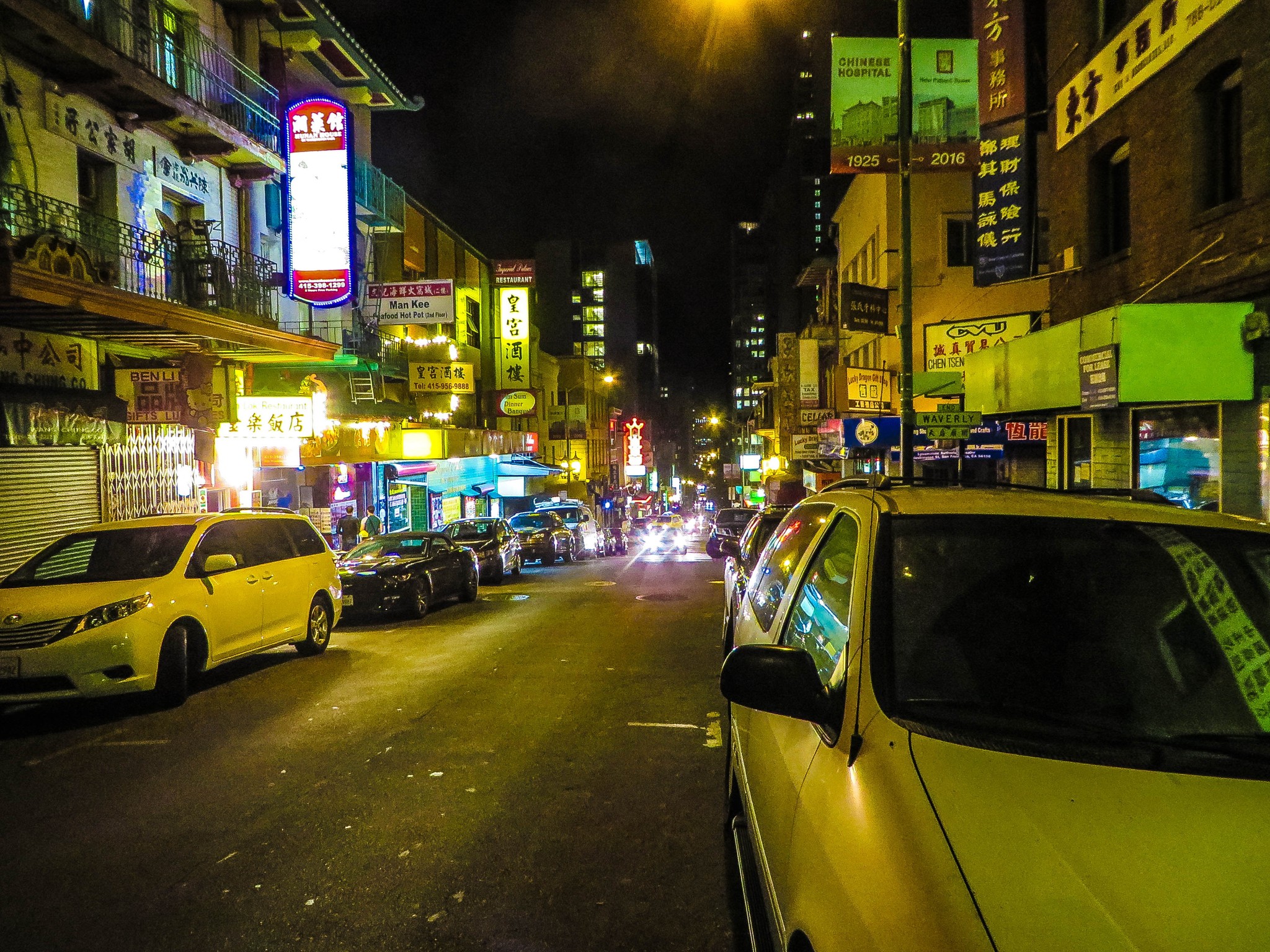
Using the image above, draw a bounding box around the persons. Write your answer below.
[336,506,360,552]
[360,505,384,545]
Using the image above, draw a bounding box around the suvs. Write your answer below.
[506,511,576,566]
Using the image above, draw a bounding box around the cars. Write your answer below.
[707,507,759,552]
[692,495,714,511]
[599,514,683,556]
[708,508,758,557]
[0,506,344,722]
[335,532,479,619]
[720,470,1270,952]
[440,517,522,585]
[719,504,795,658]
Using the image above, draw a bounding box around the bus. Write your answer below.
[533,505,598,561]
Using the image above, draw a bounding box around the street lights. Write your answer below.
[565,374,615,500]
